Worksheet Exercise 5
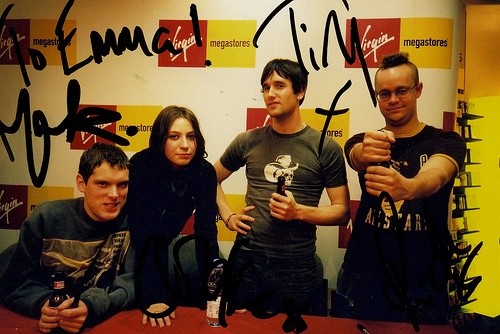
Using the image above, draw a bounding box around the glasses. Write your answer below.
[374,82,419,103]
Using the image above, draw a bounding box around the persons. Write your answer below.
[0,141,135,333]
[126,107,246,327]
[213,58,350,316]
[336,53,467,324]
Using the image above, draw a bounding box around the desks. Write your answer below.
[0,304,459,334]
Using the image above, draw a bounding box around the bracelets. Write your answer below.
[226,213,236,227]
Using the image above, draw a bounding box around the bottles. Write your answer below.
[370,130,392,202]
[48,269,69,334]
[272,176,290,243]
[207,258,226,328]
